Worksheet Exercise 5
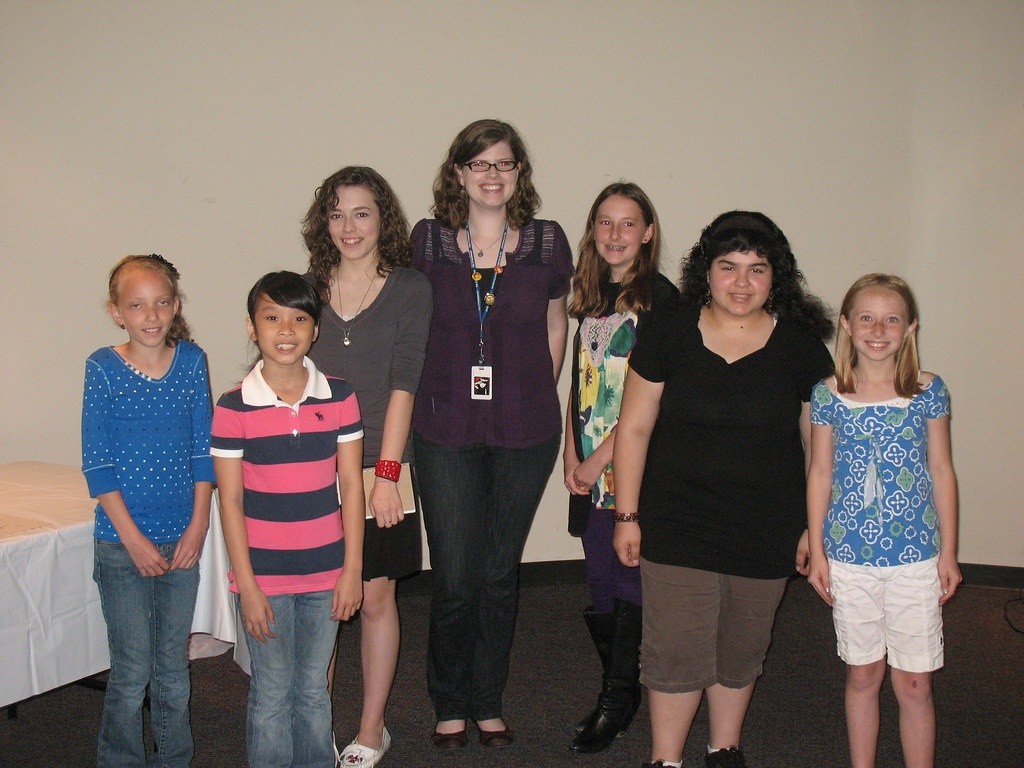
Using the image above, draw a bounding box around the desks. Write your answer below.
[0,454,257,710]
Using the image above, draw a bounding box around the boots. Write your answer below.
[567,597,642,754]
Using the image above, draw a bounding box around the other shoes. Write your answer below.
[340,726,391,768]
[474,717,513,748]
[705,747,746,768]
[433,718,468,748]
[642,760,684,768]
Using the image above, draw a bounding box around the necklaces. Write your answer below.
[336,268,378,346]
[471,233,504,257]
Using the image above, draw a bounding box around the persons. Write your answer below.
[610,210,839,768]
[210,269,365,768]
[562,182,683,755]
[81,251,217,768]
[807,272,962,768]
[297,163,433,768]
[405,119,574,751]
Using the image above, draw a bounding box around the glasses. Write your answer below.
[463,160,518,172]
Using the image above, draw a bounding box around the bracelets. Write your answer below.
[375,460,402,482]
[614,512,640,522]
